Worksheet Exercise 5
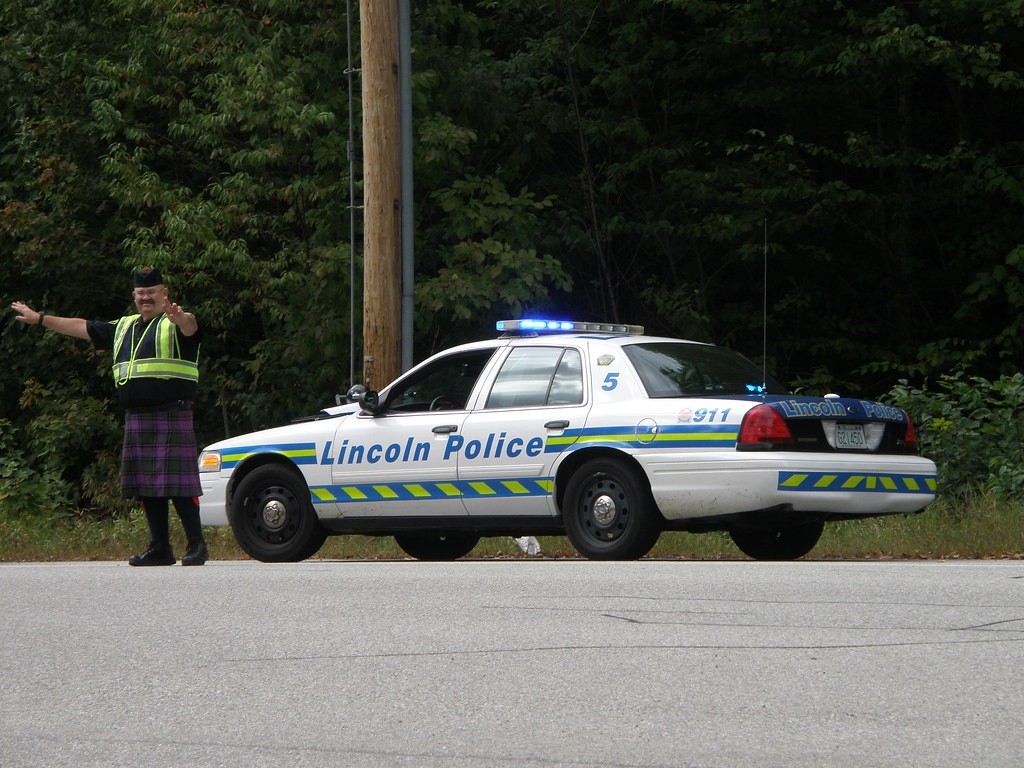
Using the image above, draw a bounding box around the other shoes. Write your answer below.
[182,542,209,566]
[129,542,176,566]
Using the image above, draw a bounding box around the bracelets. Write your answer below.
[38,311,44,325]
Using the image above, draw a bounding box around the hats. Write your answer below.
[132,267,163,287]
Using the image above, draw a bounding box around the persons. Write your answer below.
[12,268,208,566]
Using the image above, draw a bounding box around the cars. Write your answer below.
[194,318,938,564]
